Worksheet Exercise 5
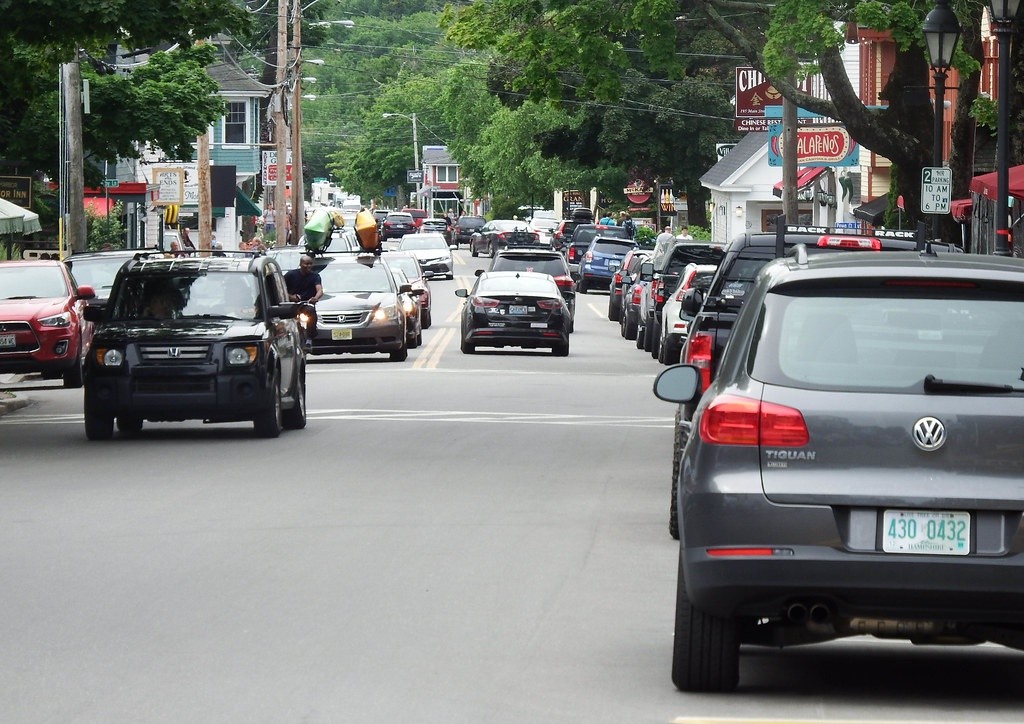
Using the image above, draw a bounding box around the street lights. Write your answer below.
[921,0,964,242]
[382,112,422,209]
[983,0,1024,256]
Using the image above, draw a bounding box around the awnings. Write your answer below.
[854,192,892,225]
[0,194,25,235]
[772,166,829,198]
[212,186,262,217]
[417,184,432,199]
[7,201,42,260]
[968,162,1024,200]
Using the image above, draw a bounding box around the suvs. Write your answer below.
[80,248,311,438]
[473,244,581,334]
[665,214,971,538]
[651,233,1024,691]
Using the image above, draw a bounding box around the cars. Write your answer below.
[452,216,488,248]
[527,205,730,365]
[455,272,577,358]
[0,260,98,389]
[261,204,458,363]
[61,246,166,315]
[469,219,542,261]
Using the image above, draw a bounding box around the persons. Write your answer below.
[262,202,276,233]
[447,208,454,221]
[285,255,324,334]
[180,227,196,250]
[512,215,518,220]
[236,236,266,258]
[598,210,636,239]
[654,226,675,248]
[676,225,693,240]
[142,290,175,320]
[169,242,185,258]
[212,241,226,258]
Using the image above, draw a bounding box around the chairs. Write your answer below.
[791,311,858,364]
[979,324,1024,377]
